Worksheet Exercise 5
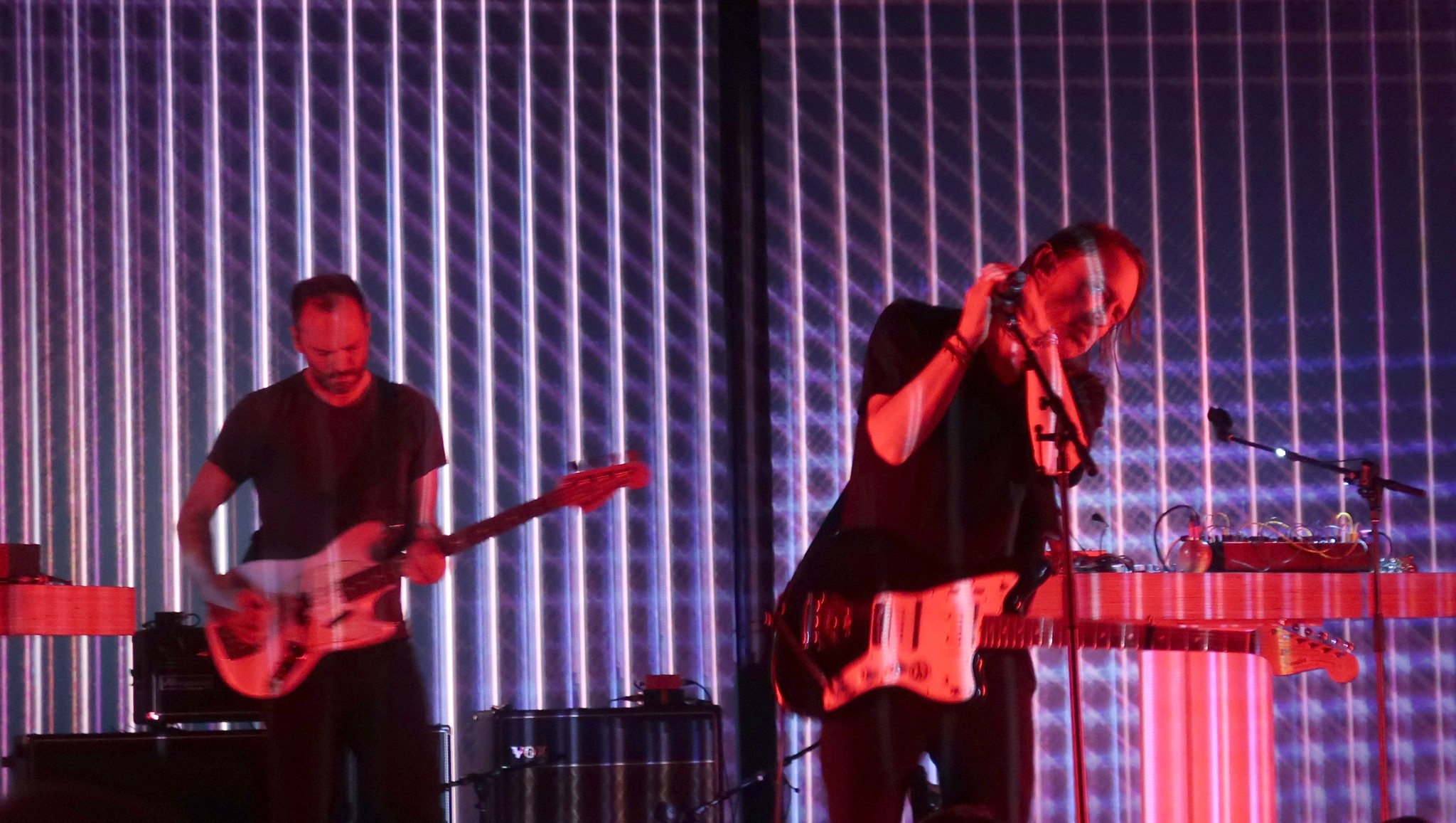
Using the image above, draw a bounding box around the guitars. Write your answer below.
[202,448,651,701]
[762,569,1360,718]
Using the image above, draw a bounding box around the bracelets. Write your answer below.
[943,331,975,365]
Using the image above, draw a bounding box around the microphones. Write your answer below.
[990,271,1027,306]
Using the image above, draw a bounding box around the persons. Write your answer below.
[781,220,1147,823]
[177,272,448,823]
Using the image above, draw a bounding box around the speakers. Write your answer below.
[6,725,452,823]
[456,709,723,823]
